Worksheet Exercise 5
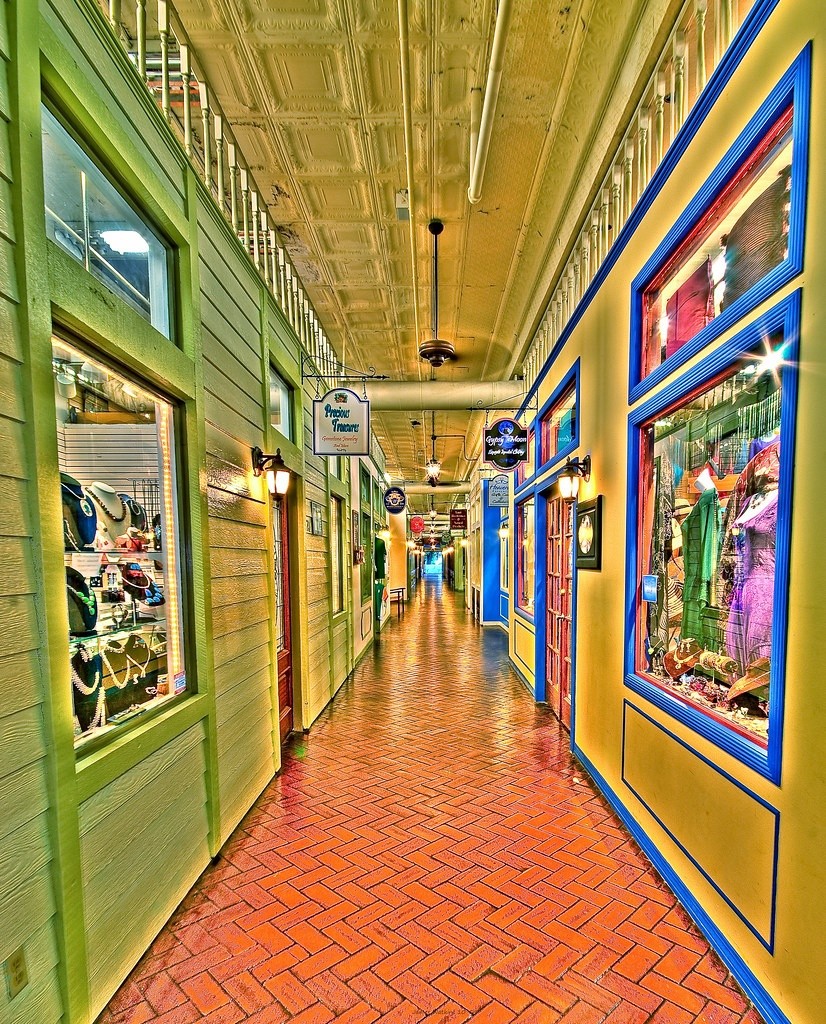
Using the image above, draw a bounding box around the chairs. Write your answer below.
[390,587,406,619]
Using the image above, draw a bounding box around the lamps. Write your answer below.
[426,409,442,487]
[417,221,455,368]
[53,357,84,398]
[429,494,437,551]
[252,446,293,507]
[557,455,591,510]
[499,522,508,542]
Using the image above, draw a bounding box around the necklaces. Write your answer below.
[751,491,770,509]
[647,636,662,655]
[729,670,770,700]
[674,647,702,669]
[60,481,164,733]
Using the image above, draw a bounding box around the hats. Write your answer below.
[668,576,684,619]
[673,499,691,517]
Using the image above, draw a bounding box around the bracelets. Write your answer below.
[699,651,739,677]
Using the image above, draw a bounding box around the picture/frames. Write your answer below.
[576,494,602,569]
[311,501,322,536]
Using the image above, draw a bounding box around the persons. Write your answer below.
[642,430,781,681]
[373,535,387,580]
